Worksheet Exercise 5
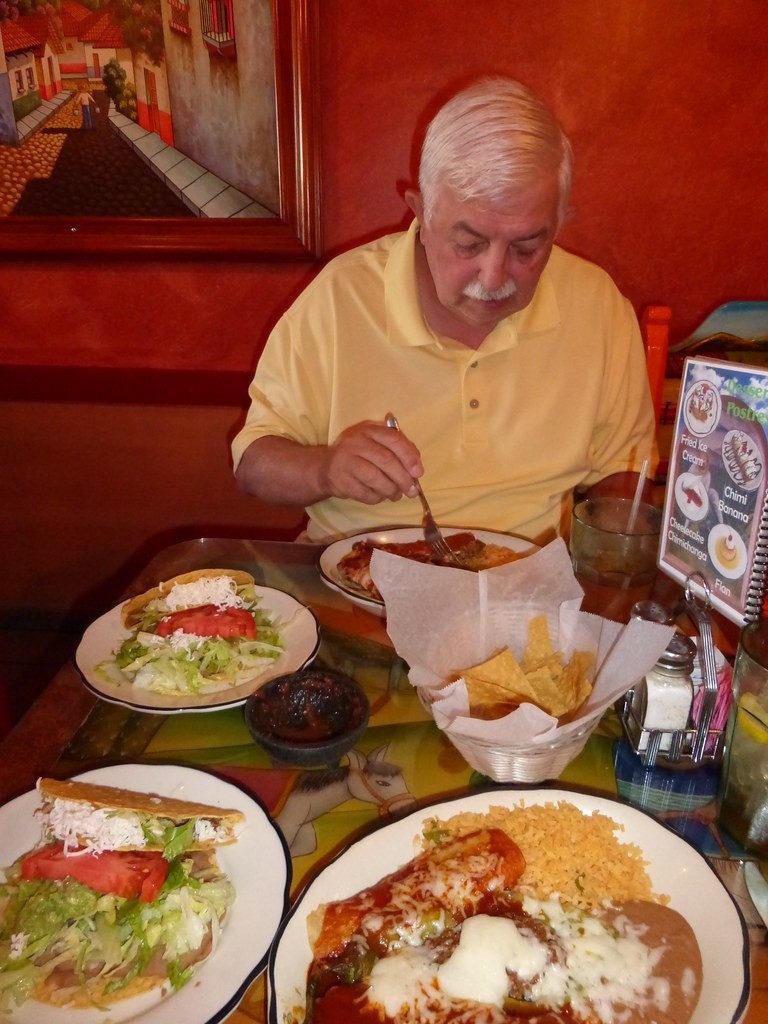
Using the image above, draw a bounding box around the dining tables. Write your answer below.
[0,535,768,1024]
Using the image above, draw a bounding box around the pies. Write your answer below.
[120,568,256,630]
[35,777,244,853]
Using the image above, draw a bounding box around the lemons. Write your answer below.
[737,691,768,746]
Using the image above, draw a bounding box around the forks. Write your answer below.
[387,417,465,569]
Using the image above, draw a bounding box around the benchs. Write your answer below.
[0,364,308,741]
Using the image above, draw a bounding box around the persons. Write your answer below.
[231,78,661,547]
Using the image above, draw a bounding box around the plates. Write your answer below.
[722,429,764,490]
[674,472,708,521]
[70,583,321,715]
[264,786,750,1024]
[316,527,543,621]
[707,523,747,579]
[0,758,293,1024]
[683,380,722,437]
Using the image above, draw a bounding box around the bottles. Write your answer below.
[627,632,697,752]
[629,598,677,629]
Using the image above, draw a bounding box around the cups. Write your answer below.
[569,497,664,624]
[717,619,768,856]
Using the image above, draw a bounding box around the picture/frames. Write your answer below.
[0,0,320,256]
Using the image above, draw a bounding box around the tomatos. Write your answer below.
[156,604,257,639]
[21,840,169,903]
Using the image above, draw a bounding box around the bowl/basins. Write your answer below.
[244,670,370,766]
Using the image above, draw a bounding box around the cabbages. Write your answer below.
[91,575,309,697]
[0,819,236,1024]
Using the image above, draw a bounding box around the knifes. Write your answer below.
[742,860,768,930]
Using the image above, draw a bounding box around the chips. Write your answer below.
[453,614,595,730]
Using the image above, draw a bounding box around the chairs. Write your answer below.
[633,306,767,510]
